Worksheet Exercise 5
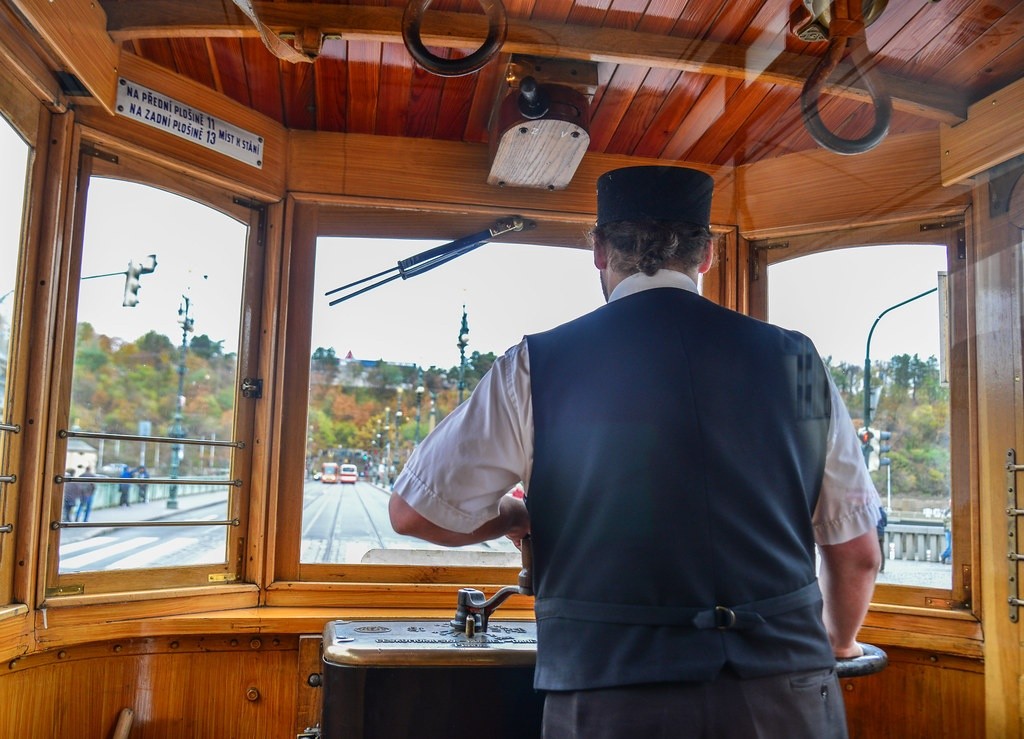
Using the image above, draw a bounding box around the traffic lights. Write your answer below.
[362,452,367,460]
[123,261,141,307]
[857,427,874,469]
[868,431,891,470]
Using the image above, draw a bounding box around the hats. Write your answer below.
[597,165,715,229]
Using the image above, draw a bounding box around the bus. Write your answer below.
[321,462,337,482]
[339,465,357,484]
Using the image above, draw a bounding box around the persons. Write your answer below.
[118,466,133,507]
[940,499,952,564]
[388,165,884,739]
[137,466,149,502]
[74,467,97,522]
[62,468,78,522]
[877,507,888,573]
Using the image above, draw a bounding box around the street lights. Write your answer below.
[371,406,390,488]
[393,399,402,483]
[166,294,194,508]
[429,394,436,434]
[414,366,425,448]
[457,305,469,405]
